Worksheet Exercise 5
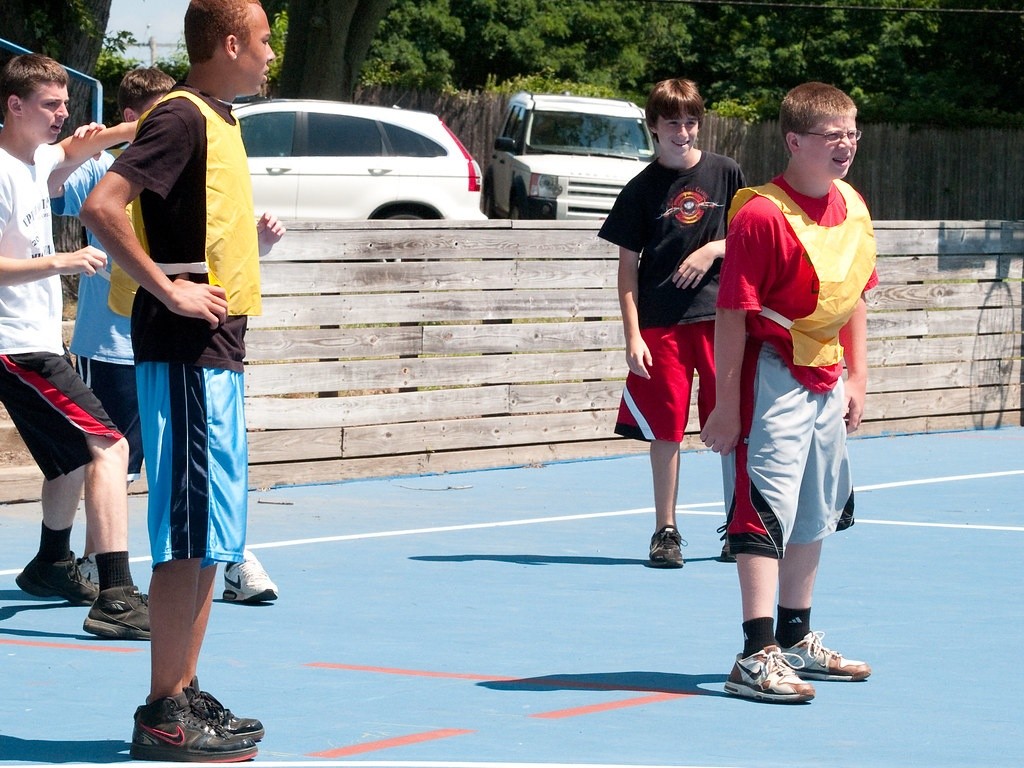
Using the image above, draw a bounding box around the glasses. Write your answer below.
[800,130,863,143]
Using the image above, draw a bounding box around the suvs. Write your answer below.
[484,89,657,220]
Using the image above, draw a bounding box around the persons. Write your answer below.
[0,53,151,641]
[79,0,277,762]
[48,68,287,602]
[701,82,879,702]
[597,78,747,566]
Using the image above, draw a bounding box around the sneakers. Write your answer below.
[717,525,737,562]
[182,676,264,741]
[130,691,258,762]
[223,549,278,602]
[76,552,100,583]
[82,584,151,640]
[724,644,815,703]
[15,550,100,606]
[771,631,871,682]
[649,524,688,567]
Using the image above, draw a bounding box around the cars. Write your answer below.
[119,97,489,262]
